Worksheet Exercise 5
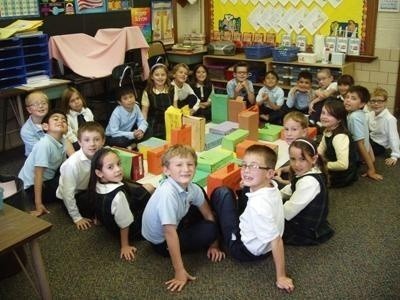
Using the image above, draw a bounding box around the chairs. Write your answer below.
[146,39,171,70]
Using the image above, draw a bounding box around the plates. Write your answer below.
[171,47,192,50]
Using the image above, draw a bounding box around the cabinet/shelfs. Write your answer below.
[166,50,206,65]
[267,60,355,100]
[0,34,54,89]
[203,53,273,94]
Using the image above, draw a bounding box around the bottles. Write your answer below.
[321,47,329,64]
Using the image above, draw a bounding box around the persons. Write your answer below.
[20,91,50,156]
[279,138,335,246]
[17,109,67,217]
[61,87,94,151]
[141,144,226,292]
[87,148,154,261]
[55,121,106,231]
[105,63,400,188]
[210,144,295,294]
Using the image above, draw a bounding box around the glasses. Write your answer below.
[368,99,385,104]
[238,163,269,170]
[26,100,49,107]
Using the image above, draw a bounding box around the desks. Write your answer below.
[0,204,52,297]
[15,78,71,127]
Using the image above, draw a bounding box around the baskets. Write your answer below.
[272,47,300,62]
[243,45,272,59]
